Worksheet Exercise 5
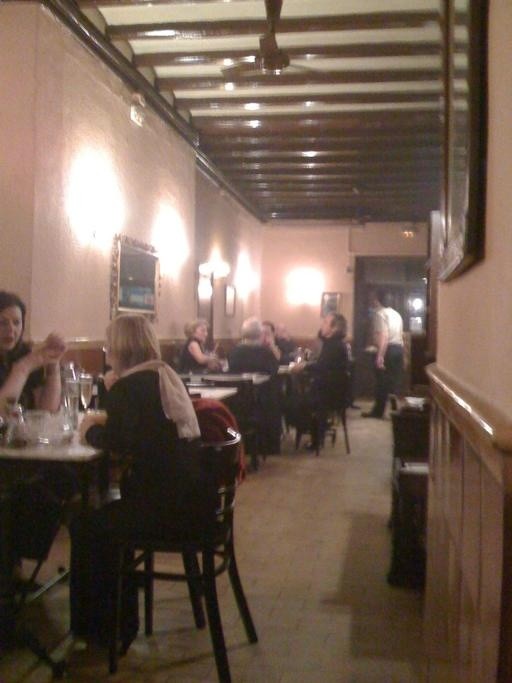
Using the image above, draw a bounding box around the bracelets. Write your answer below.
[46,370,60,375]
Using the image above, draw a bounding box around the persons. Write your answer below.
[260,320,293,366]
[176,317,223,373]
[288,311,349,451]
[359,286,405,418]
[59,311,208,653]
[226,317,284,457]
[2,289,68,594]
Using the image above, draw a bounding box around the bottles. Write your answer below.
[8,403,29,447]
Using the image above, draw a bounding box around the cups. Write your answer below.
[63,378,81,422]
[26,408,51,446]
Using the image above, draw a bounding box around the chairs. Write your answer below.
[1,344,356,480]
[99,426,259,680]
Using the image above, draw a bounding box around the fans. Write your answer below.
[212,0,333,83]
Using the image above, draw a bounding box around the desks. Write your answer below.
[1,401,115,680]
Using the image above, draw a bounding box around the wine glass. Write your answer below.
[79,374,95,413]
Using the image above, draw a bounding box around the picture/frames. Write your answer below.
[223,284,236,319]
[433,2,488,279]
[319,291,341,320]
[111,233,161,326]
